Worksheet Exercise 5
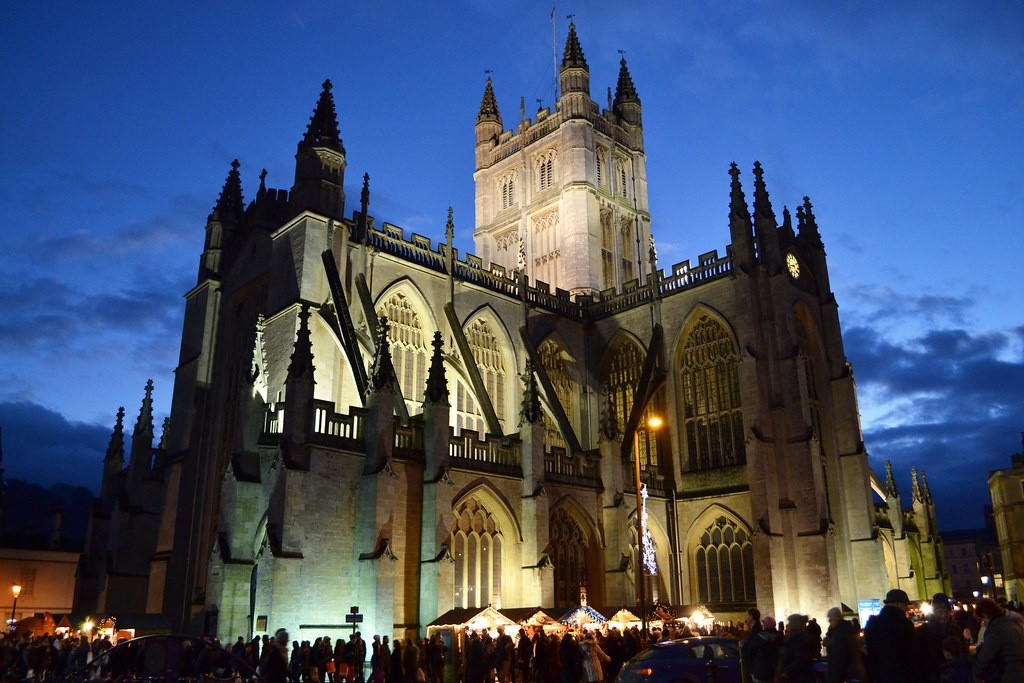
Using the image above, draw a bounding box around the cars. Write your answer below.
[619,635,833,682]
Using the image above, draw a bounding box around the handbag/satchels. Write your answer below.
[338,663,348,677]
[325,661,335,674]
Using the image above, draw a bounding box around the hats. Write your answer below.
[787,614,808,629]
[883,589,913,606]
[761,616,776,630]
[828,608,842,620]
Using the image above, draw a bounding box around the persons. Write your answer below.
[0,588,1024,683]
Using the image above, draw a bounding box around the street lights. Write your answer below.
[631,412,666,650]
[9,583,21,632]
